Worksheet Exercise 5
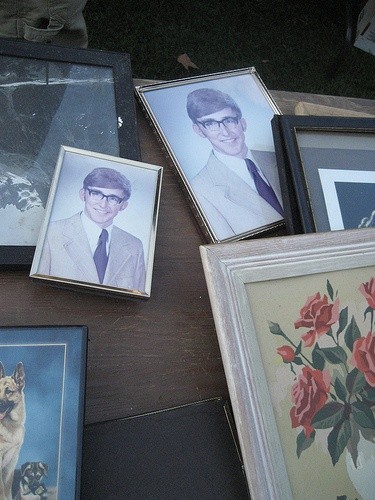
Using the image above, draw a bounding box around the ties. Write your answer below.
[243,158,288,221]
[92,229,110,285]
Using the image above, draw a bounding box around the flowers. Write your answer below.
[269,274,375,471]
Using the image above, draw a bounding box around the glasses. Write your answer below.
[87,187,125,206]
[194,115,245,131]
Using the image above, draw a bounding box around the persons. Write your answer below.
[81,0,204,79]
[35,166,147,292]
[186,88,288,241]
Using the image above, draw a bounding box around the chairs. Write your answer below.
[324,0,357,79]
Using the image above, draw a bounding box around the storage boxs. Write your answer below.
[347,0,375,56]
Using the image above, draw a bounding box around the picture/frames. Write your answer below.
[270,114,375,236]
[200,227,375,500]
[133,66,287,246]
[0,323,90,500]
[82,396,252,500]
[0,35,143,267]
[28,145,164,302]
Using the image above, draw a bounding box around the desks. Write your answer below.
[1,76,374,500]
